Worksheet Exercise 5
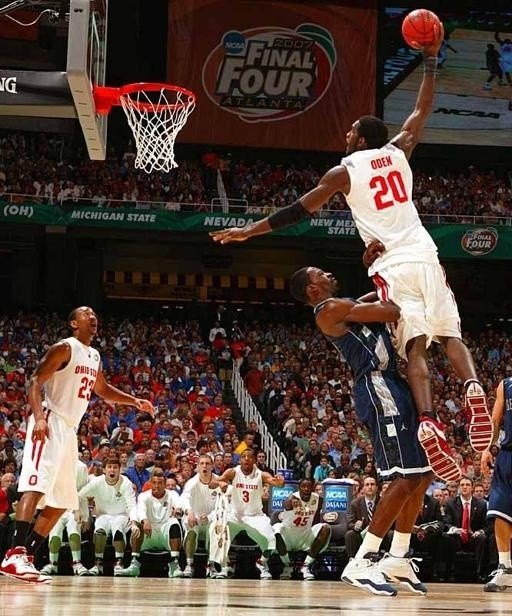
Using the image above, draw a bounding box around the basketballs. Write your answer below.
[402,9,440,49]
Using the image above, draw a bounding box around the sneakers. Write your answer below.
[483,564,512,593]
[463,379,494,454]
[2,545,316,583]
[377,552,428,594]
[418,417,461,482]
[341,557,398,595]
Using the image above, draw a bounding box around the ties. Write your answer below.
[462,502,470,543]
[367,502,374,524]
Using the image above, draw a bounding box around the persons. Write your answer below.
[290,239,434,596]
[209,22,494,483]
[1,129,512,225]
[2,306,512,584]
[481,378,511,593]
